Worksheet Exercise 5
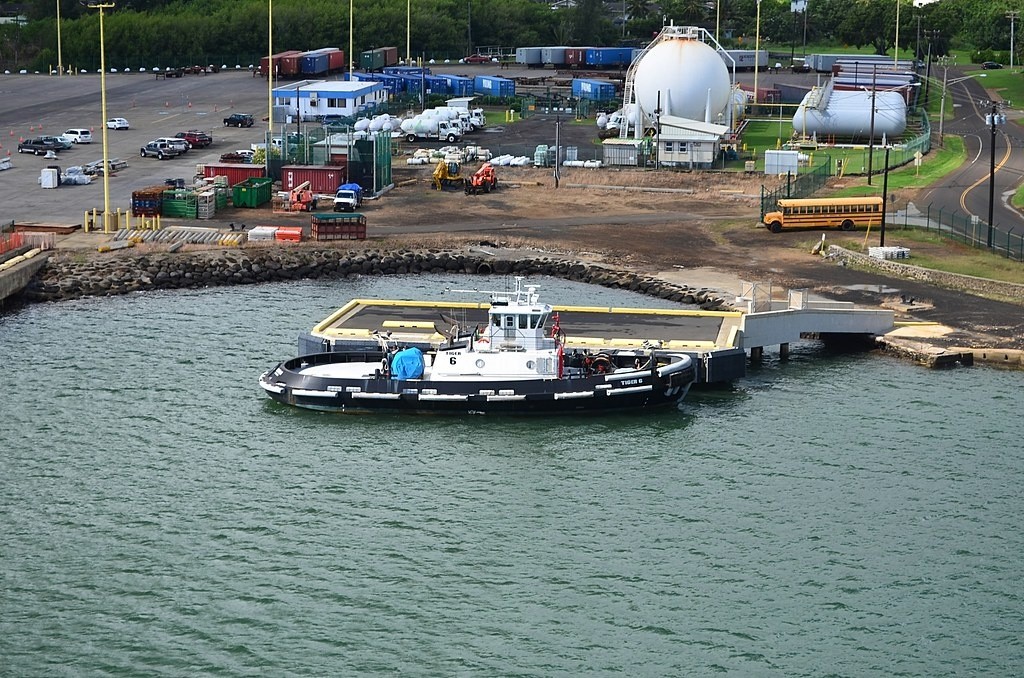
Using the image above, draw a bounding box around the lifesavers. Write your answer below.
[478,339,489,343]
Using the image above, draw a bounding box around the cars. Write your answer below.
[62,129,92,144]
[106,117,129,130]
[464,54,493,66]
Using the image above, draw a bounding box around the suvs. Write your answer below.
[981,60,1003,70]
[224,113,256,129]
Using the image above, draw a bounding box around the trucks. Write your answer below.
[400,108,486,142]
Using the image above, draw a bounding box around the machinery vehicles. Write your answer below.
[430,161,500,197]
[282,182,316,211]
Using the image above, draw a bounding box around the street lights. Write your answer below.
[87,2,116,233]
[977,99,1012,249]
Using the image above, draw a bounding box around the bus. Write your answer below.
[762,199,883,234]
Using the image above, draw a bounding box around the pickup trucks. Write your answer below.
[140,130,212,160]
[333,187,363,212]
[18,135,73,156]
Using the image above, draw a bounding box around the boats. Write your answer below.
[258,276,695,419]
